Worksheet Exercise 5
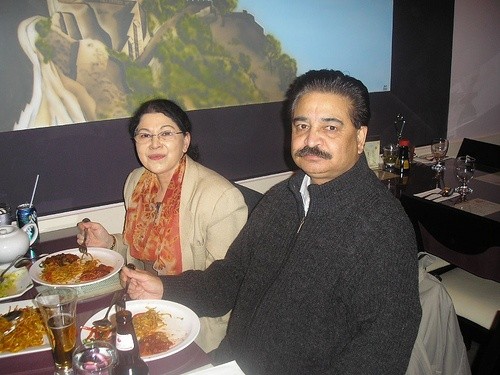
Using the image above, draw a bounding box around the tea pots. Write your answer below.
[0,222,38,269]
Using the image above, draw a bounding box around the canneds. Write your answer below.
[16,203,40,244]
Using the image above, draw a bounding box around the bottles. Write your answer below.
[113,311,150,375]
[399,140,410,172]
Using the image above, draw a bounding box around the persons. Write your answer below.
[77,98,249,355]
[120,70,423,375]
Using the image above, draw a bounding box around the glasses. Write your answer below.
[133,131,185,144]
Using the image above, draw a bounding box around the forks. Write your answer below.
[93,295,115,328]
[79,218,90,256]
[115,264,136,312]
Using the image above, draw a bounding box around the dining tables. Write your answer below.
[0,234,216,375]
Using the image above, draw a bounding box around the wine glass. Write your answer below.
[455,157,476,195]
[431,137,449,169]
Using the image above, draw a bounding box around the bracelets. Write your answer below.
[110,235,116,250]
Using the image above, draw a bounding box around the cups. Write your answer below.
[382,144,398,168]
[73,341,119,375]
[34,287,78,375]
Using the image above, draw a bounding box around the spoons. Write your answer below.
[422,187,454,199]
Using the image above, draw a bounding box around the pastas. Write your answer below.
[40,258,101,284]
[130,307,171,337]
[0,304,56,356]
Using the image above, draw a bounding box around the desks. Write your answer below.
[359,152,500,253]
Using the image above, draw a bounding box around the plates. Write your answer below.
[0,261,33,301]
[80,299,200,362]
[29,247,124,287]
[0,294,61,357]
